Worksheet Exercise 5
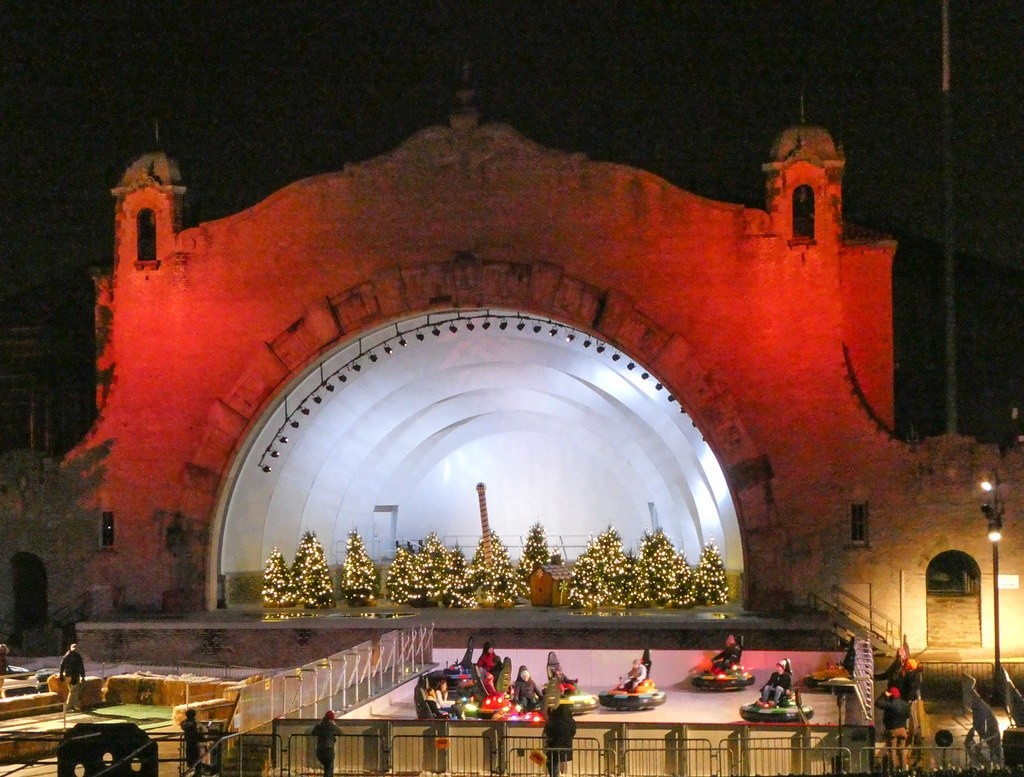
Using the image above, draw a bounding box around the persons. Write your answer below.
[619,659,647,692]
[543,703,577,777]
[704,634,741,675]
[180,709,217,768]
[311,710,343,777]
[476,641,511,696]
[513,670,543,710]
[59,644,85,713]
[426,679,466,720]
[553,666,578,696]
[0,644,10,699]
[756,660,790,709]
[873,647,921,771]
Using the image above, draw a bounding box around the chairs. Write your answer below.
[542,679,559,718]
[471,664,489,697]
[495,657,512,692]
[641,649,651,677]
[734,635,744,663]
[547,652,560,679]
[461,637,475,665]
[415,674,433,719]
[784,657,792,674]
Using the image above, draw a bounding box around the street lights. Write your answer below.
[979,467,1005,705]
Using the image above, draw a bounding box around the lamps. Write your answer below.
[258,317,706,474]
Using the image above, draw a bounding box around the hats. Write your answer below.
[777,660,787,670]
[325,711,335,719]
[726,635,735,644]
[890,688,901,698]
[521,670,530,676]
[905,659,920,670]
